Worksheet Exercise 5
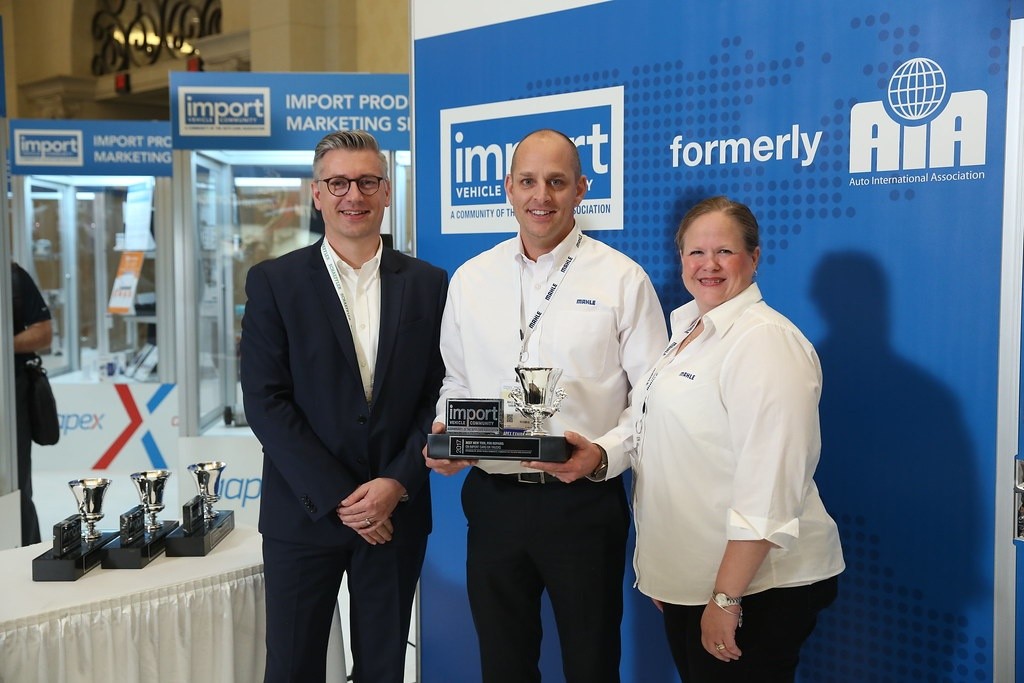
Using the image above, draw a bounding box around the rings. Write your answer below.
[552,472,556,477]
[366,518,371,525]
[716,644,725,650]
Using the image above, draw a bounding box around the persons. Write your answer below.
[630,196,845,683]
[422,129,670,683]
[10,261,61,547]
[241,133,449,683]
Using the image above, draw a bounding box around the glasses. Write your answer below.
[317,175,384,197]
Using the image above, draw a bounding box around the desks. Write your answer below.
[0,526,348,683]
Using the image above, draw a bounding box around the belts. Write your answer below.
[508,472,562,483]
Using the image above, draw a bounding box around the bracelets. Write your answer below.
[711,596,737,615]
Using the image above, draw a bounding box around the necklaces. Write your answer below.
[685,335,692,345]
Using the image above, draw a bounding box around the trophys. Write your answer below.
[31,478,121,582]
[165,461,234,557]
[101,470,179,569]
[427,367,572,463]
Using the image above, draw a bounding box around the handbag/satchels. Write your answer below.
[26,363,60,446]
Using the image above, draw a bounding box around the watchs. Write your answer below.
[590,444,607,479]
[711,590,742,608]
